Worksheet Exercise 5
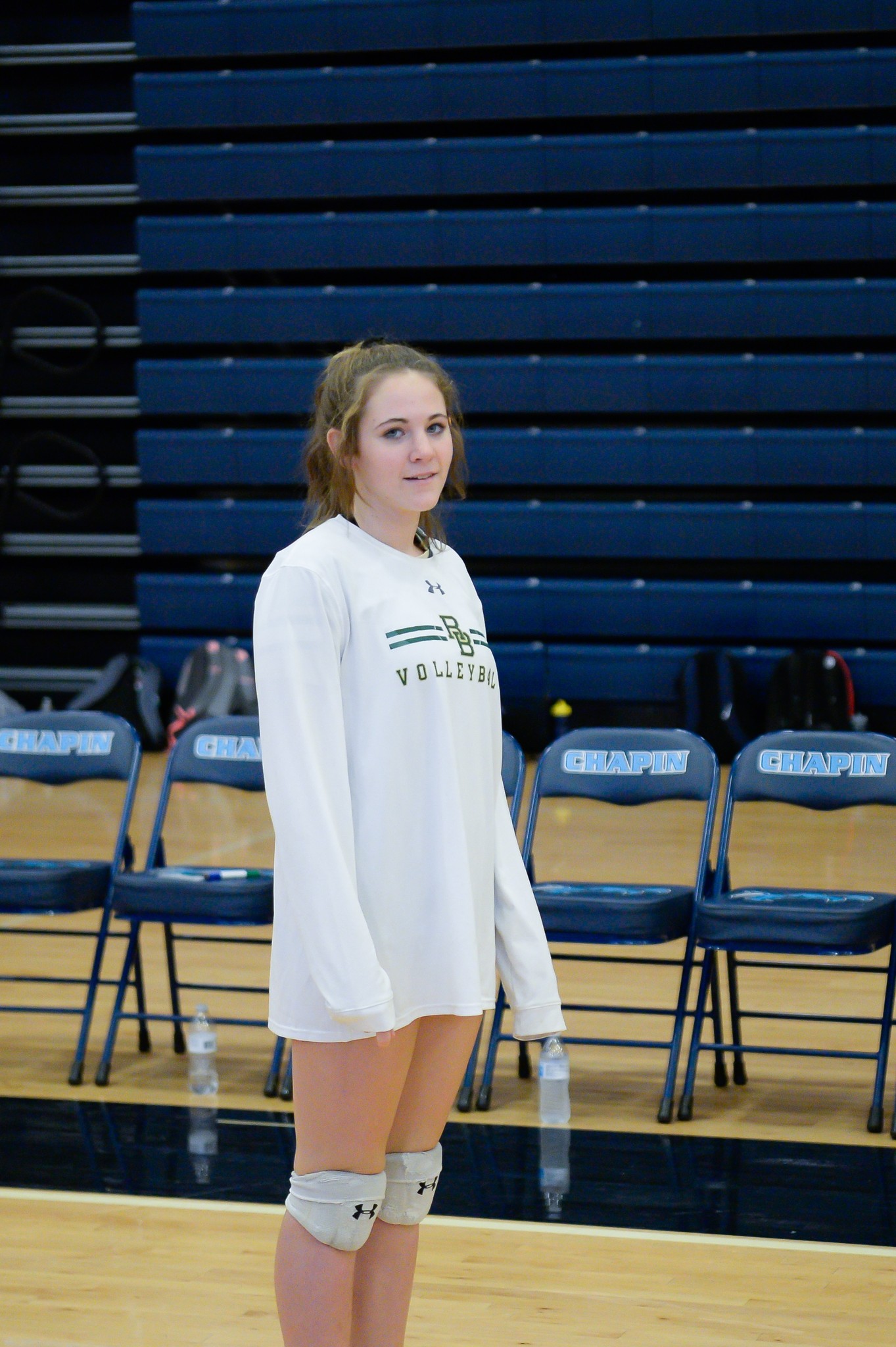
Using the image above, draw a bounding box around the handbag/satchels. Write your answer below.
[499,697,572,754]
[566,695,684,730]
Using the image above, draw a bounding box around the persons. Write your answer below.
[253,342,567,1347]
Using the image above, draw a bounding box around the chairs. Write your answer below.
[475,726,728,1122]
[96,715,284,1098]
[280,731,526,1111]
[678,728,896,1133]
[0,710,150,1083]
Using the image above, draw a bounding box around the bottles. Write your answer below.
[538,1034,571,1124]
[189,1107,221,1182]
[187,1004,219,1095]
[538,1130,571,1213]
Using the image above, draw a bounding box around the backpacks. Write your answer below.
[165,638,259,749]
[673,646,763,766]
[763,647,867,736]
[68,652,171,750]
